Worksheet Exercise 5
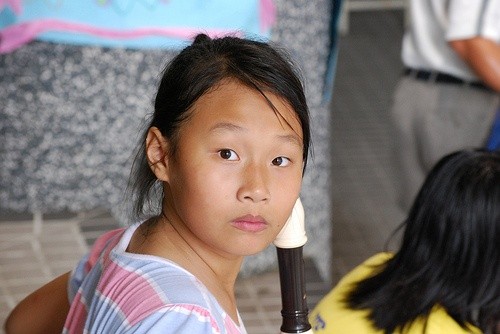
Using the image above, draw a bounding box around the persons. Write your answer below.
[3,34,315,334]
[391,0,500,222]
[307,148,500,334]
